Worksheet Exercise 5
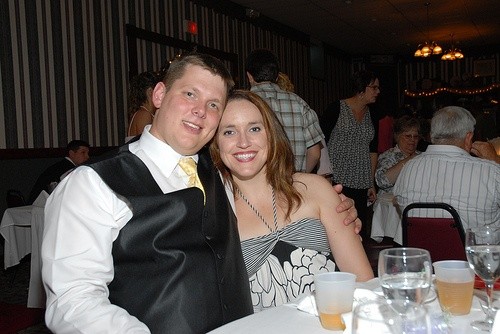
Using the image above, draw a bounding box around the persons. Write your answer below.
[207,88,376,315]
[374,115,423,194]
[123,70,164,148]
[41,50,363,334]
[319,68,381,226]
[242,47,326,174]
[275,72,333,179]
[26,139,90,205]
[393,105,499,246]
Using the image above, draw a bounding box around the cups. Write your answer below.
[432,259,476,316]
[313,271,358,330]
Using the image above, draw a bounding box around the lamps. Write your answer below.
[413,39,442,58]
[440,46,464,61]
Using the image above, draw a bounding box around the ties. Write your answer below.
[178,157,207,206]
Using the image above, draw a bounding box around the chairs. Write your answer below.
[401,201,469,274]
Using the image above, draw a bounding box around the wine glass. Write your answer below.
[464,223,499,333]
[378,247,432,334]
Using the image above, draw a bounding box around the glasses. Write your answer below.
[399,134,421,139]
[364,84,381,91]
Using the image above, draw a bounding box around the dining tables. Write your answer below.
[207,273,500,334]
